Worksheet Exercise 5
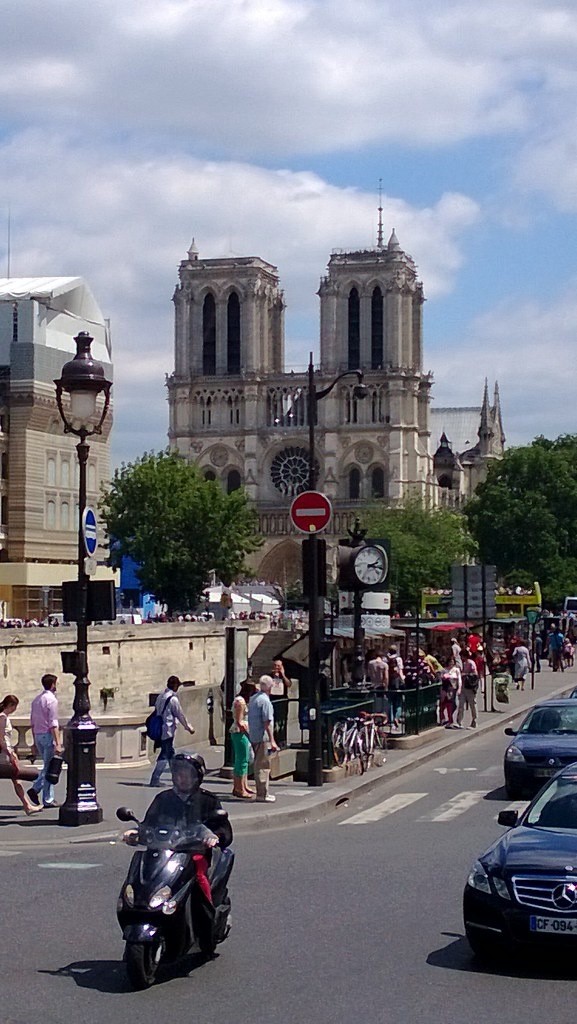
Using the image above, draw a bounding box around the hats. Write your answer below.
[168,675,183,684]
[389,644,397,651]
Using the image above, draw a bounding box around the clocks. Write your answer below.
[353,544,388,586]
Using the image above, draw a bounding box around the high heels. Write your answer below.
[26,804,44,816]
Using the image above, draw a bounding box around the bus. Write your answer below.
[421,582,542,619]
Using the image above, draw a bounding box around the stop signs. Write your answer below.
[289,490,332,535]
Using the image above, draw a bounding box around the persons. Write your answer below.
[404,611,412,617]
[120,750,232,910]
[254,655,292,752]
[0,694,44,817]
[507,622,573,691]
[247,674,281,802]
[368,651,389,728]
[228,680,256,798]
[26,673,64,808]
[391,611,400,618]
[0,609,310,633]
[387,648,405,728]
[437,632,485,730]
[148,675,195,787]
[432,608,438,618]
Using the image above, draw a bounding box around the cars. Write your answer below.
[504,686,577,798]
[463,762,577,955]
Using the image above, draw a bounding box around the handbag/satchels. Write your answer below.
[45,753,64,784]
[464,673,479,689]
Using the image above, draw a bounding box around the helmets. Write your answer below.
[171,751,206,794]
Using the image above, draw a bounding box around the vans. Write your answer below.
[564,596,577,613]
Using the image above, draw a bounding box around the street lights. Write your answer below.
[52,331,114,826]
[308,352,369,786]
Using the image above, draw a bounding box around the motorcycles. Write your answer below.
[116,758,234,989]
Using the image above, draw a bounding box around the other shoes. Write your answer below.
[151,782,166,787]
[394,718,399,728]
[453,722,465,729]
[445,721,454,728]
[27,789,40,805]
[245,787,257,794]
[43,800,64,807]
[438,718,446,726]
[233,789,253,798]
[466,726,477,730]
[256,794,277,802]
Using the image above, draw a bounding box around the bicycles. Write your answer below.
[331,710,388,774]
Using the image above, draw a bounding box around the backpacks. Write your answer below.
[146,695,173,741]
[385,656,399,679]
